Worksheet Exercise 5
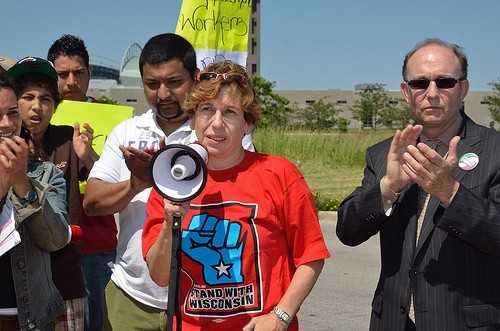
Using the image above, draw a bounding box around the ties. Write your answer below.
[406,139,441,325]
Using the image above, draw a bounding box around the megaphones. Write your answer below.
[149,141,210,202]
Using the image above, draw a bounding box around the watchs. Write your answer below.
[21,190,38,203]
[272,307,293,325]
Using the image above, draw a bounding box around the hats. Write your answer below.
[8,56,58,85]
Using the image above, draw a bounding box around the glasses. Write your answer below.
[196,71,252,86]
[403,77,464,90]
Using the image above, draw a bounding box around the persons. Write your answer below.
[8,56,88,331]
[336,38,500,331]
[47,34,119,331]
[144,60,330,331]
[0,64,72,331]
[83,34,259,331]
